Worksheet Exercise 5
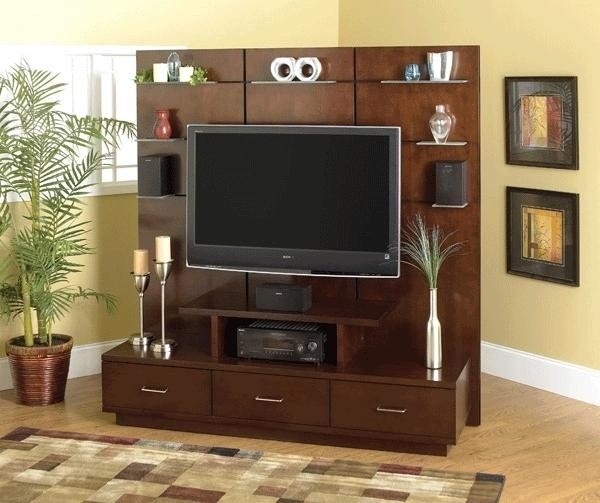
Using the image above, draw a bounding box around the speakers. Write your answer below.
[435,159,467,205]
[138,153,174,197]
[256,282,313,312]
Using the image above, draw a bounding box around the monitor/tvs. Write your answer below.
[185,123,402,278]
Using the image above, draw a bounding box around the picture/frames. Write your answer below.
[504,76,579,169]
[506,186,579,288]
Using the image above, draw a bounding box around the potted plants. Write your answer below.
[378,213,472,368]
[0,60,136,405]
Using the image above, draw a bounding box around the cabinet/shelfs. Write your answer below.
[136,46,480,366]
[103,337,472,458]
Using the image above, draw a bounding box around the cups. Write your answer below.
[428,51,453,81]
[153,63,169,82]
[405,64,421,81]
[179,67,193,82]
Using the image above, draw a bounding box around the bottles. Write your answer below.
[430,104,451,143]
[154,110,171,138]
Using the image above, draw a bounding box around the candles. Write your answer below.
[154,235,171,260]
[134,249,149,274]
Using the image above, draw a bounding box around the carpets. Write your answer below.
[1,426,505,502]
